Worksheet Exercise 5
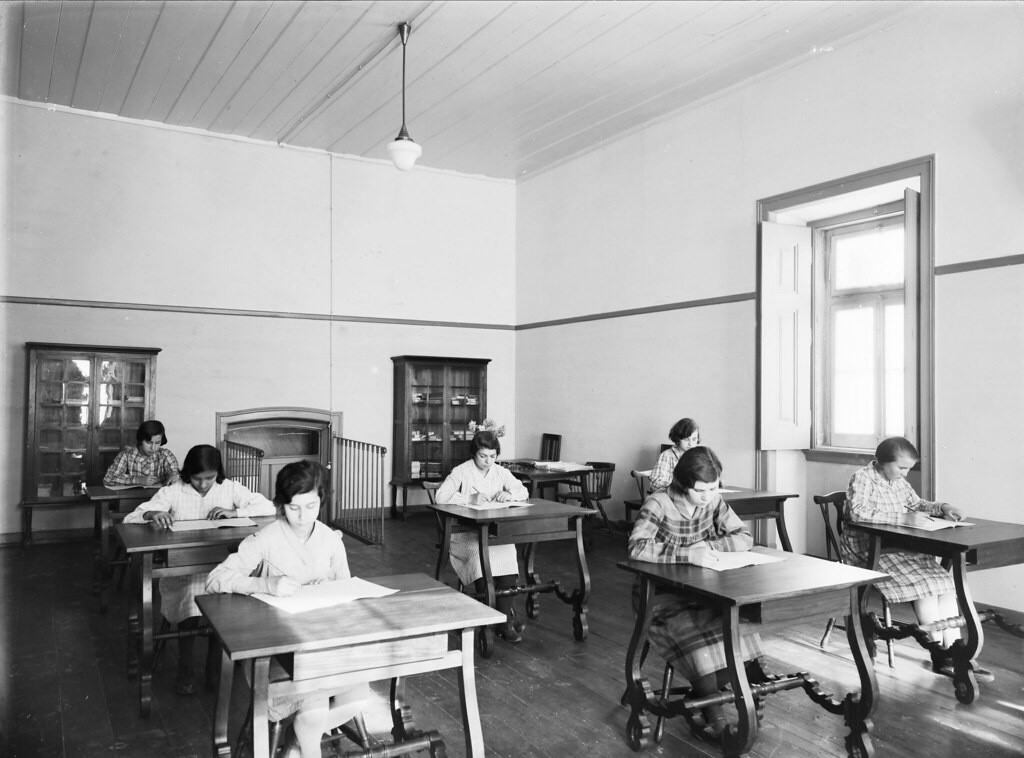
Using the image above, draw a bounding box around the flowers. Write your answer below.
[468,418,507,440]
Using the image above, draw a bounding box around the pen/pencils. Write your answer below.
[263,558,286,576]
[472,486,491,503]
[707,538,719,561]
[904,505,934,522]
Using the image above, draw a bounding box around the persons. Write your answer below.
[205,460,351,758]
[627,446,766,737]
[649,417,723,492]
[840,437,995,684]
[102,420,180,487]
[435,432,529,643]
[121,444,275,696]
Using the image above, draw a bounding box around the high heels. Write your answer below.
[496,623,522,642]
[510,618,526,633]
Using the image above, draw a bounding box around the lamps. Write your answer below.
[387,21,423,172]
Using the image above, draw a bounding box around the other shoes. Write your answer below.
[205,669,220,693]
[174,664,195,694]
[941,659,994,682]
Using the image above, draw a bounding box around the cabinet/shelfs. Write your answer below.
[18,344,163,550]
[388,355,493,520]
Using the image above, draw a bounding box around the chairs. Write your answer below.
[557,462,616,546]
[422,481,527,633]
[523,433,563,502]
[814,491,897,668]
[623,443,674,541]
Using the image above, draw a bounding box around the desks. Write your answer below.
[426,498,600,660]
[113,515,278,682]
[495,458,599,553]
[616,544,893,758]
[719,485,800,553]
[84,485,162,602]
[848,516,1024,705]
[197,571,508,758]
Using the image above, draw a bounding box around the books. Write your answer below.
[534,461,594,473]
[411,392,479,479]
[38,482,86,497]
[59,395,143,404]
